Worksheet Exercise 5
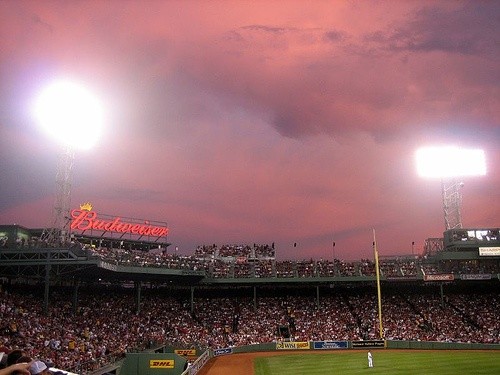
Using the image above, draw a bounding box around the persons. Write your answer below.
[195,242,500,343]
[0,250,212,375]
[367,349,374,367]
